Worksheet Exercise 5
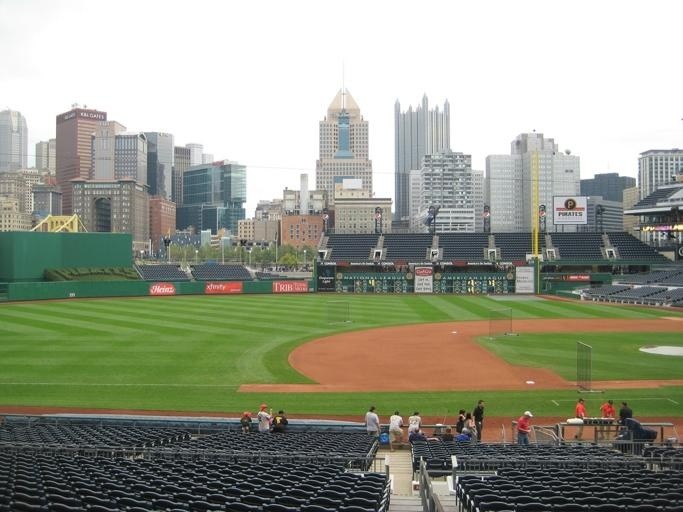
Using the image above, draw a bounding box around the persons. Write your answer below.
[365,406,380,437]
[443,400,485,443]
[614,402,632,437]
[598,400,615,440]
[663,232,668,241]
[257,404,288,434]
[409,427,439,443]
[388,410,404,452]
[517,411,533,445]
[240,412,252,433]
[574,398,586,440]
[622,417,641,430]
[408,412,422,439]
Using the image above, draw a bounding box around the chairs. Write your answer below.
[133,262,272,282]
[626,185,683,209]
[410,434,683,512]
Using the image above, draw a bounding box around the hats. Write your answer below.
[524,410,533,418]
[259,403,268,412]
[242,411,252,417]
